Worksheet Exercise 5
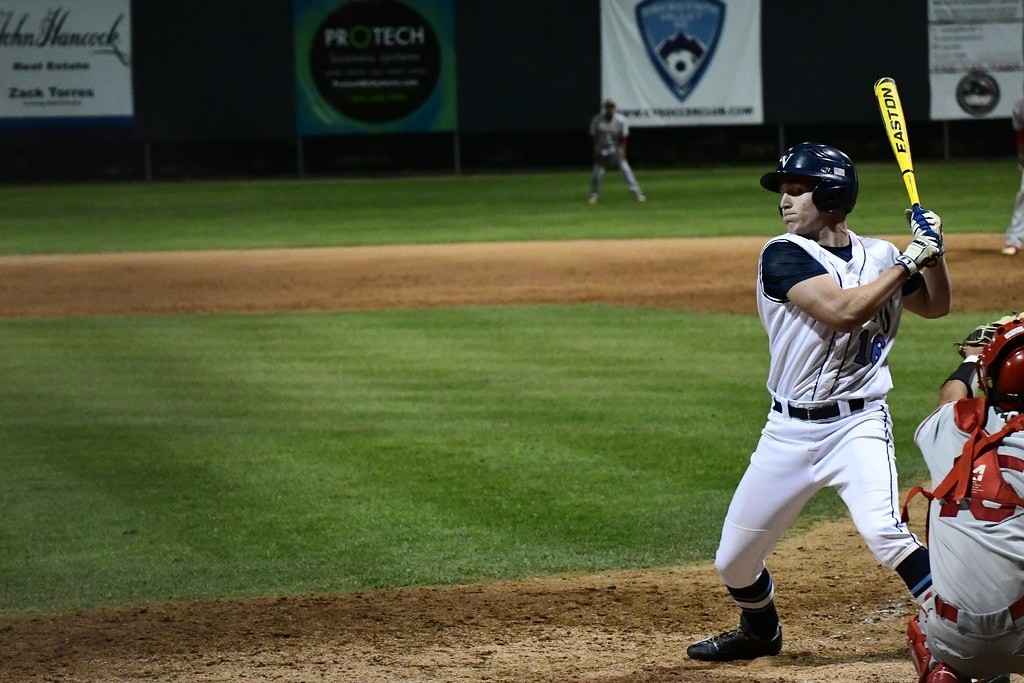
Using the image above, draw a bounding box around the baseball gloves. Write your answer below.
[959,312,1024,357]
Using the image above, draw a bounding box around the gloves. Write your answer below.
[894,208,945,277]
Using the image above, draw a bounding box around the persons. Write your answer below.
[1000,85,1024,256]
[587,97,647,205]
[686,141,1012,683]
[900,313,1024,683]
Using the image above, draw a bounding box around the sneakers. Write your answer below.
[687,623,782,661]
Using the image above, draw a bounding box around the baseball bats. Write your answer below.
[873,76,939,268]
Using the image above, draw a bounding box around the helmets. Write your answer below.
[976,318,1024,410]
[760,141,859,212]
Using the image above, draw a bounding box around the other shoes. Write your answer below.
[1001,246,1017,255]
[924,662,1011,683]
[633,193,645,202]
[587,195,598,204]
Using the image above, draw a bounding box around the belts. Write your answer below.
[925,592,1024,624]
[773,397,864,420]
